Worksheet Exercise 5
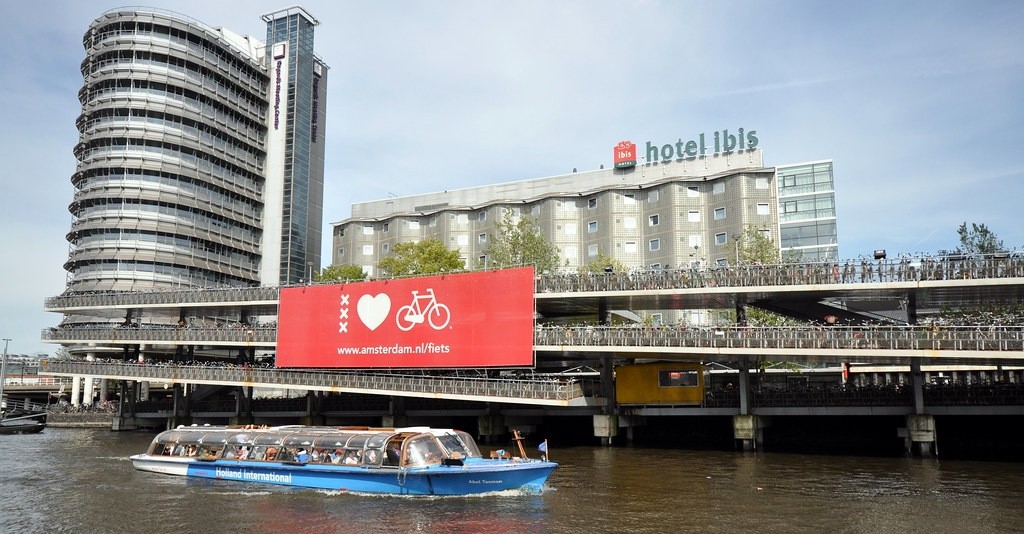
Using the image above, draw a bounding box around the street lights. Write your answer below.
[307,261,314,284]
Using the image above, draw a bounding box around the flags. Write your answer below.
[538,442,546,453]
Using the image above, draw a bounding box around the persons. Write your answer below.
[535,315,1024,351]
[47,357,275,369]
[164,443,432,466]
[537,249,1024,293]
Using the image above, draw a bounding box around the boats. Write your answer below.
[128,424,558,496]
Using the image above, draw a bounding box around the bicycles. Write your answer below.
[535,316,1024,351]
[535,248,1024,293]
[527,375,578,399]
[708,375,1024,406]
[57,401,120,414]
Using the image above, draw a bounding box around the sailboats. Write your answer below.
[0,348,49,438]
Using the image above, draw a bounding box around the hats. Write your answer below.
[298,448,304,452]
[335,449,343,454]
